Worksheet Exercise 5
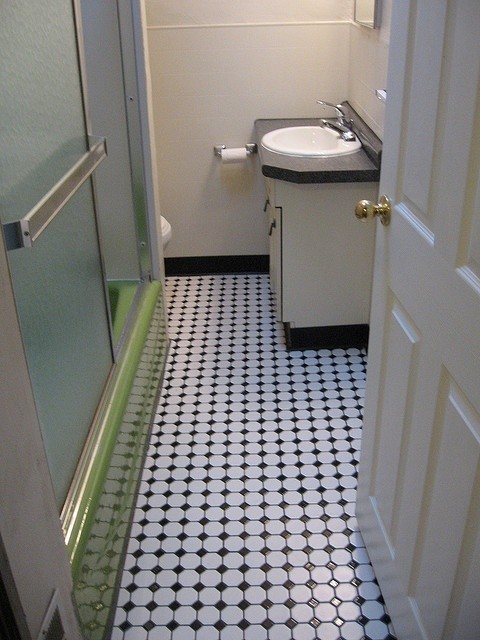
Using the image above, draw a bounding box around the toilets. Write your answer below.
[159,215,171,254]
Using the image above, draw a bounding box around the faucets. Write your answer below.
[316,99,356,141]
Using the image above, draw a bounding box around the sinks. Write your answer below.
[264,127,343,155]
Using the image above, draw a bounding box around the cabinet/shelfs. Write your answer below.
[261,176,375,355]
